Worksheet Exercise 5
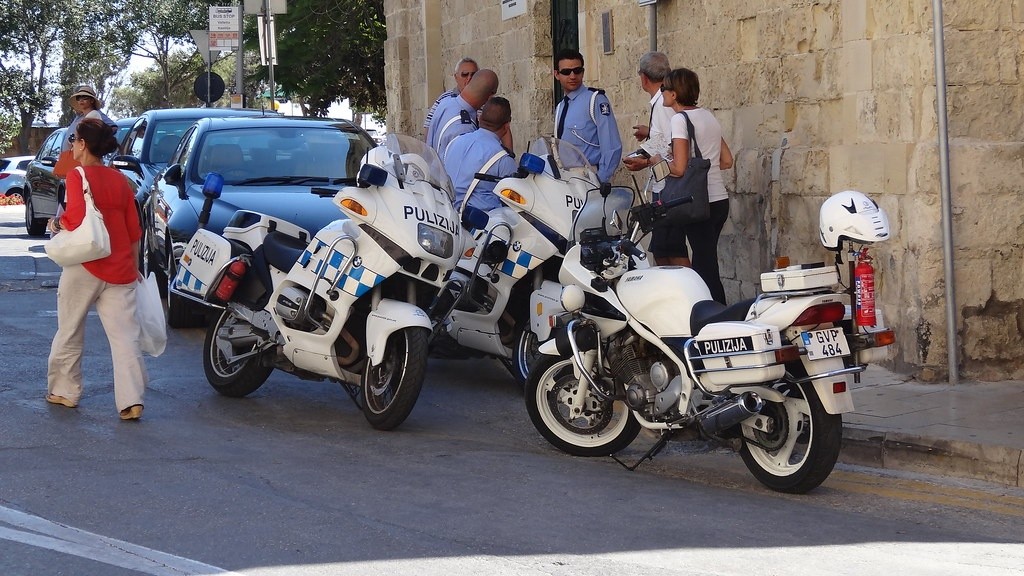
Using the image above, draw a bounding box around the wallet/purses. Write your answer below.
[626,148,652,161]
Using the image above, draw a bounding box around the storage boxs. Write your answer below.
[853,309,888,362]
[760,267,838,292]
[695,320,786,387]
[173,230,253,304]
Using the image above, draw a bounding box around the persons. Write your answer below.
[622,51,733,307]
[423,57,519,218]
[46,86,118,237]
[44,119,150,419]
[552,49,623,184]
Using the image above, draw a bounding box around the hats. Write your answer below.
[69,86,101,111]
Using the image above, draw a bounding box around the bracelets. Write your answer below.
[647,157,652,168]
[50,217,62,234]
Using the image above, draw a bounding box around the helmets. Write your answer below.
[818,190,891,250]
[356,144,388,187]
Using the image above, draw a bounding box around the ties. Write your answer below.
[557,96,570,137]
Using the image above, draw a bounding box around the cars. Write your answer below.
[108,107,281,235]
[23,126,106,236]
[143,115,379,328]
[102,116,139,167]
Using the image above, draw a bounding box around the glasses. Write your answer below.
[660,83,675,92]
[461,71,476,78]
[638,70,642,76]
[68,133,82,143]
[558,66,583,75]
[75,94,90,101]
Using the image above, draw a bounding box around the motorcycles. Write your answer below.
[525,175,896,494]
[169,133,489,430]
[429,135,611,391]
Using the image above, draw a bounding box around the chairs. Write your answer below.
[155,135,185,163]
[208,143,311,175]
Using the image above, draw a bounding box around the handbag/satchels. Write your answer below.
[656,111,713,221]
[44,166,112,267]
[136,271,167,358]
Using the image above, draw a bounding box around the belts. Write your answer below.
[559,166,598,176]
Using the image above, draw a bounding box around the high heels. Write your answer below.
[46,394,76,407]
[119,403,143,419]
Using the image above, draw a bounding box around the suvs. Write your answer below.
[0,155,36,204]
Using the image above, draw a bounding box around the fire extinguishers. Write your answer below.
[216,253,252,302]
[854,247,877,328]
